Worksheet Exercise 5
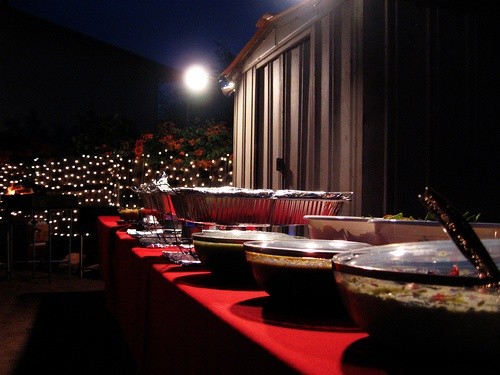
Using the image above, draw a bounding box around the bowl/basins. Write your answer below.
[192,233,295,280]
[331,239,500,358]
[243,239,372,303]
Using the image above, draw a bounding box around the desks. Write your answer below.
[98,215,500,374]
